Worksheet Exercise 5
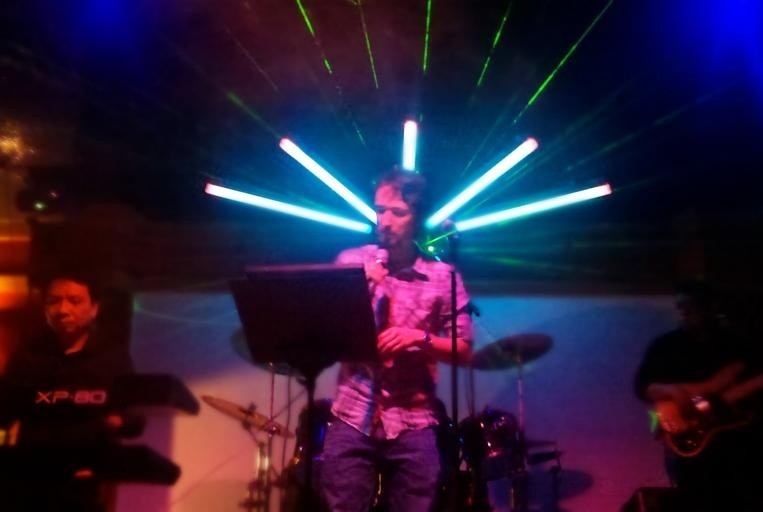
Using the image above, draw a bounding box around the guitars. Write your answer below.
[657,363,761,457]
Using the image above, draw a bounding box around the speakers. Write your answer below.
[620,486,685,511]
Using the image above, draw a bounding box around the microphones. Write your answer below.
[369,248,390,293]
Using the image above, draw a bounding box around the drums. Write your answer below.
[296,398,332,454]
[460,408,521,480]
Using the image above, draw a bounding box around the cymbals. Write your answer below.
[201,395,292,439]
[471,334,553,370]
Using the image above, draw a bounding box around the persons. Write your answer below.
[635,286,761,510]
[0,273,147,509]
[321,173,473,511]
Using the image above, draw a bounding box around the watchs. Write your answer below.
[421,328,431,349]
